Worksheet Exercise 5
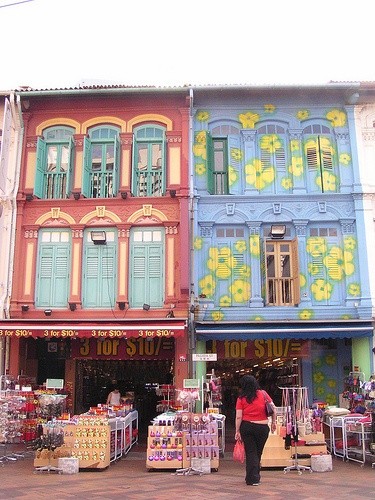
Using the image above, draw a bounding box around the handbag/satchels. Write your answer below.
[233,440,245,463]
[280,388,321,450]
[260,390,274,417]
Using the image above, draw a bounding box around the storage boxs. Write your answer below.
[192,457,211,475]
[311,455,332,472]
[59,457,80,474]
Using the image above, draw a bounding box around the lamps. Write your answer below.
[26,190,176,202]
[90,231,106,245]
[21,303,197,315]
[271,225,286,239]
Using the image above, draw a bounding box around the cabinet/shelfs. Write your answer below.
[108,410,139,464]
[260,434,327,467]
[151,412,227,455]
[320,414,375,468]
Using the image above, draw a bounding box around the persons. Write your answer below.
[234,375,277,486]
[107,388,121,407]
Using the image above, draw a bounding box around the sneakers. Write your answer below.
[252,480,262,486]
